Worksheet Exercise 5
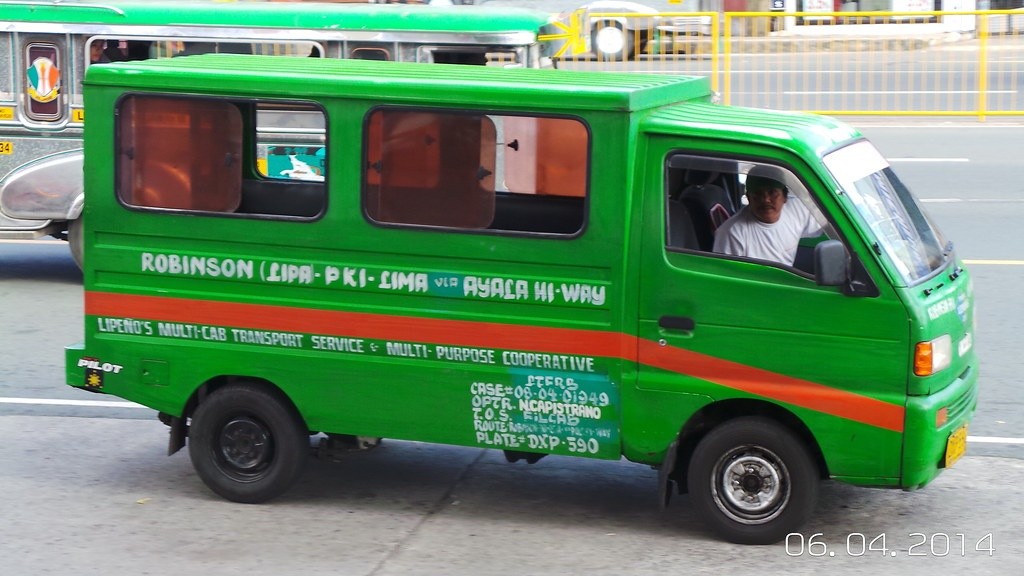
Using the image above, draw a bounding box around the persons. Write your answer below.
[90,39,128,64]
[713,166,827,264]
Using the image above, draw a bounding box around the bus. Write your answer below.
[0,1,559,275]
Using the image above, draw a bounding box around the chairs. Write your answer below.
[679,183,732,252]
[669,199,702,251]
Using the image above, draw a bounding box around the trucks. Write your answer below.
[63,51,981,545]
[359,2,662,61]
